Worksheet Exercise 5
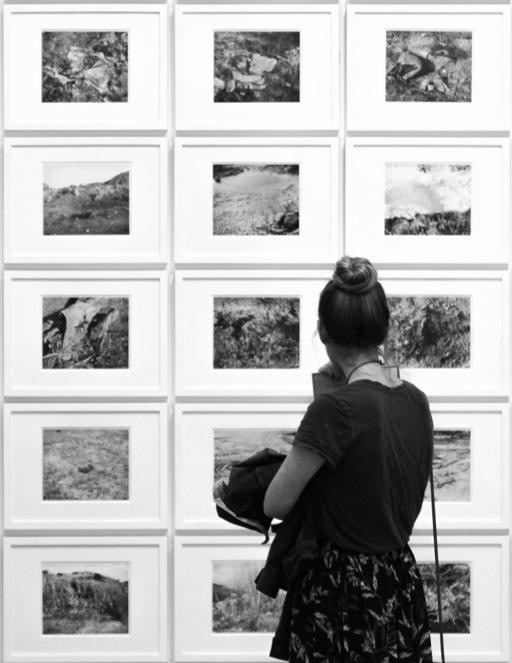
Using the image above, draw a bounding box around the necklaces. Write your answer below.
[346,358,383,384]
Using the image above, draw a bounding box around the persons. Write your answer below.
[260,256,440,662]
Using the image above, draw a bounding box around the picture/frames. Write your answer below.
[2,404,171,536]
[170,2,343,137]
[411,405,509,538]
[343,2,510,136]
[346,137,510,271]
[0,136,171,269]
[170,138,345,269]
[369,280,511,405]
[2,536,170,663]
[171,406,312,533]
[171,270,333,403]
[2,267,171,404]
[412,537,509,663]
[0,1,170,137]
[171,534,290,660]
